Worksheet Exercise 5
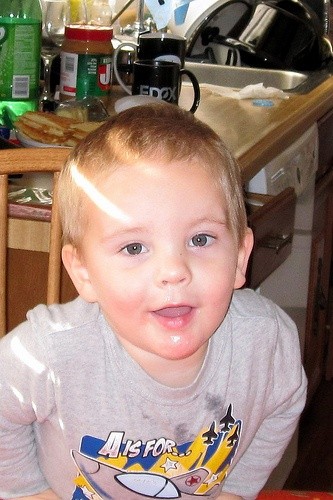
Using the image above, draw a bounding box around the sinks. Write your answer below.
[185,55,333,74]
[180,60,323,96]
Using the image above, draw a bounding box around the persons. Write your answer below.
[0,101,308,500]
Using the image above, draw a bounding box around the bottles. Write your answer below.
[0,0,43,129]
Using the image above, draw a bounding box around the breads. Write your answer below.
[13,109,108,148]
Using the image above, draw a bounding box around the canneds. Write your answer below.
[59,24,114,108]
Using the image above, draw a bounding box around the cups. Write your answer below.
[132,60,201,114]
[46,0,167,51]
[113,33,187,96]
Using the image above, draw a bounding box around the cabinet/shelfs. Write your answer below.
[6,110,333,409]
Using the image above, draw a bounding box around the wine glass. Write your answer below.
[39,0,69,106]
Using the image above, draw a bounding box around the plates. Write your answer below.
[15,129,72,148]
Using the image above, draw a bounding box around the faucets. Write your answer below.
[134,0,165,44]
